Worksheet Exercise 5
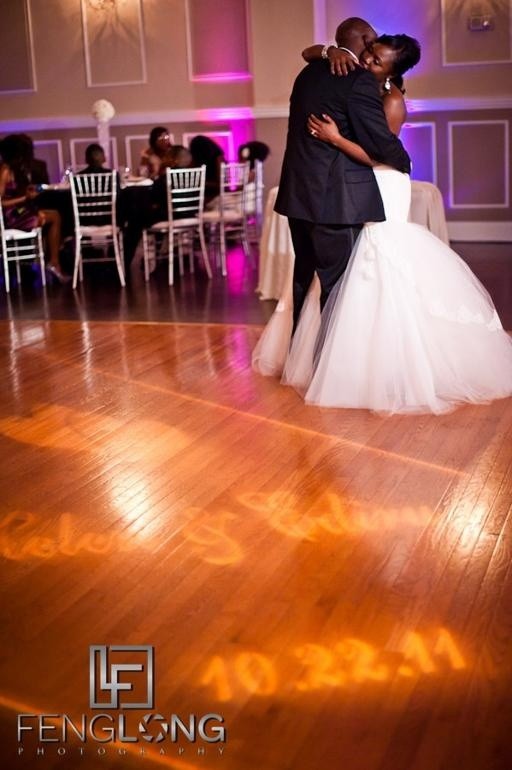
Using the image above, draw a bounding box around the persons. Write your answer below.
[0,126,225,296]
[271,16,412,378]
[251,31,512,414]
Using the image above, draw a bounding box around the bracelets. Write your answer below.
[321,45,334,61]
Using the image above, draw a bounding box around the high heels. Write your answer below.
[46,263,73,285]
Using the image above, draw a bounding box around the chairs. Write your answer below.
[1,159,264,292]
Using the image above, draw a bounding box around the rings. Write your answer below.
[310,130,316,135]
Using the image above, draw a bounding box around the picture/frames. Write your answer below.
[441,0,512,68]
[0,0,38,96]
[81,0,147,88]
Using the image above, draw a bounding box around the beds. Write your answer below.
[256,180,449,302]
